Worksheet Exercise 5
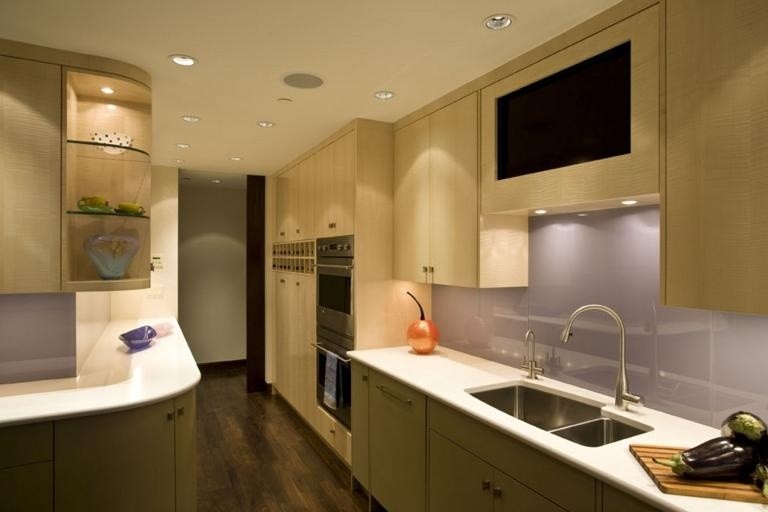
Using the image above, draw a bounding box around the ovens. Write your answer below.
[310,235,355,432]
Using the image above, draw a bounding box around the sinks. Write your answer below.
[470,384,603,431]
[549,418,646,448]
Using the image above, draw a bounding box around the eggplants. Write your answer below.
[722,410,767,465]
[650,437,754,483]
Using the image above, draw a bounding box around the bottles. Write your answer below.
[84,233,140,279]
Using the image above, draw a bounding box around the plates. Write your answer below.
[90,132,134,156]
[112,209,147,217]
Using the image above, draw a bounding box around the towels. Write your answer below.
[324,351,338,410]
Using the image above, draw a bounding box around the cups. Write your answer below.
[117,202,143,213]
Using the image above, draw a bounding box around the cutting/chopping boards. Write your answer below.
[628,442,767,506]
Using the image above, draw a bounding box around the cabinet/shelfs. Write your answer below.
[658,0,768,317]
[62,50,152,291]
[595,479,662,511]
[393,79,529,289]
[271,272,316,434]
[275,118,394,239]
[314,405,352,471]
[369,368,426,512]
[53,389,196,512]
[426,396,595,512]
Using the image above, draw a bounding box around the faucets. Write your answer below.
[521,330,544,379]
[558,305,641,409]
